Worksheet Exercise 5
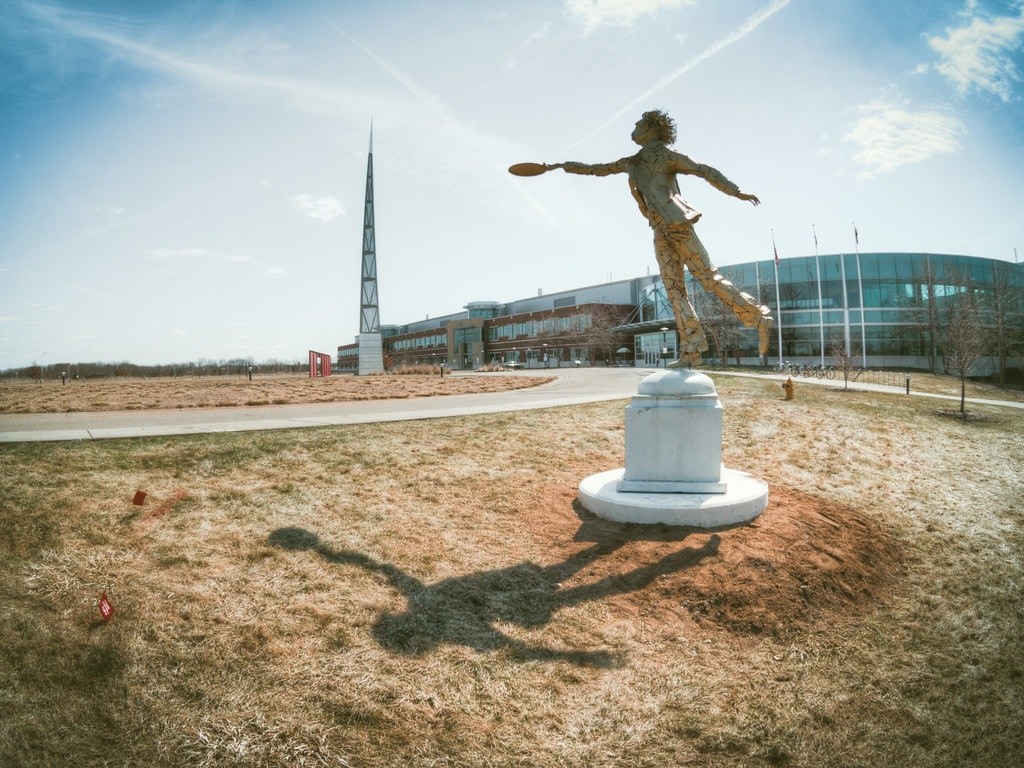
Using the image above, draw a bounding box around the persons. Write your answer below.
[542,110,774,369]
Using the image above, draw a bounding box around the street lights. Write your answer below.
[543,344,549,368]
[384,355,388,369]
[481,350,484,367]
[512,347,516,360]
[660,327,669,369]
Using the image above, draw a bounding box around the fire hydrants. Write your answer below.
[782,378,794,400]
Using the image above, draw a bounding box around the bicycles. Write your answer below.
[774,361,835,380]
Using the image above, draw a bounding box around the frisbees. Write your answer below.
[507,162,544,177]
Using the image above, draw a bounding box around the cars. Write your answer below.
[502,360,523,370]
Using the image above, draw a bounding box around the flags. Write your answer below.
[773,240,779,266]
[853,227,860,243]
[814,234,819,250]
